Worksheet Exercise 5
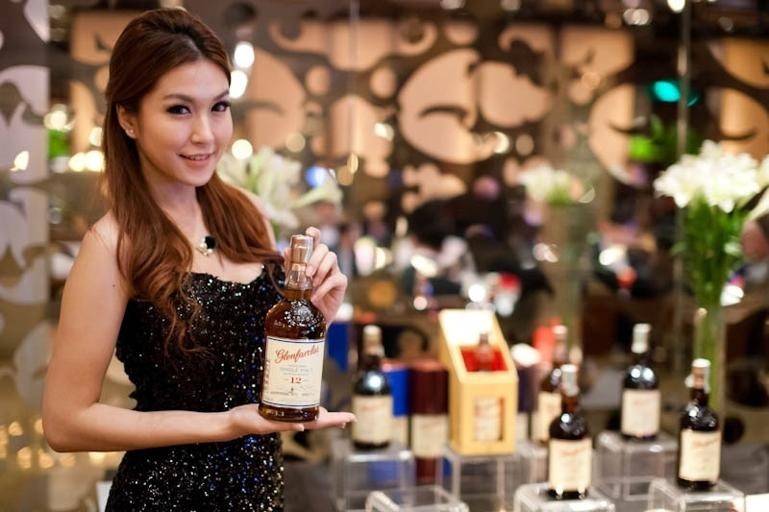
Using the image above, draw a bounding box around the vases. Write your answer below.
[691,307,725,437]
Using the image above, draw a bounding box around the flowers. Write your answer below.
[652,147,769,417]
[216,150,343,240]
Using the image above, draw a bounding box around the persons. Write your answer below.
[40,6,360,512]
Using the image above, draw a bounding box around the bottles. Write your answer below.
[549,364,593,501]
[538,325,572,444]
[676,358,722,490]
[473,327,503,441]
[351,324,395,450]
[259,234,327,421]
[620,322,661,440]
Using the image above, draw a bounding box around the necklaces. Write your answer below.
[190,236,219,256]
